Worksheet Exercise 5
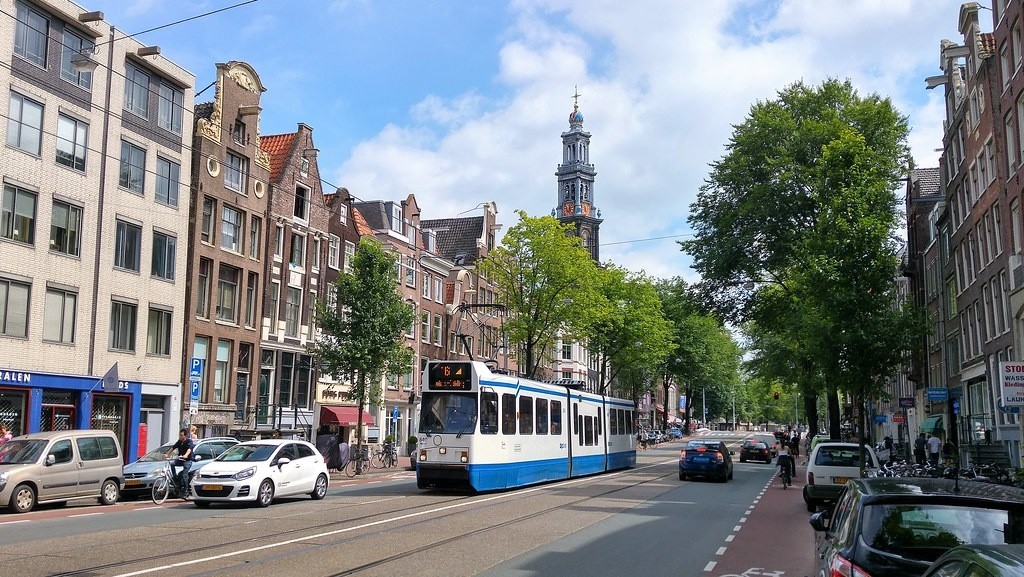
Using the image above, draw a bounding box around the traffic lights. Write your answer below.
[774,392,779,400]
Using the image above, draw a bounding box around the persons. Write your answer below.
[0,425,13,462]
[881,436,896,451]
[165,429,194,499]
[914,433,928,465]
[927,432,942,466]
[943,438,955,466]
[776,426,801,485]
[641,429,648,445]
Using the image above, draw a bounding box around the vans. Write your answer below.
[0,429,127,513]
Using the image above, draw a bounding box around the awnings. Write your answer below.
[921,417,943,432]
[320,406,374,426]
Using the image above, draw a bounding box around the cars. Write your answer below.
[184,438,330,507]
[638,428,683,447]
[679,440,735,483]
[920,543,1024,577]
[738,427,788,465]
[410,448,416,470]
[809,474,1024,577]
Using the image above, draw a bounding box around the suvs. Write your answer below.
[802,432,880,512]
[119,436,242,501]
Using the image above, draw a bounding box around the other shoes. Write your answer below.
[181,491,190,498]
[779,472,782,477]
[170,478,177,484]
[788,483,792,486]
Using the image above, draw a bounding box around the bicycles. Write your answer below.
[780,468,791,491]
[861,459,1013,485]
[152,455,196,505]
[371,442,399,470]
[345,445,375,478]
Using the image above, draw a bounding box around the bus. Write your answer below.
[417,302,638,492]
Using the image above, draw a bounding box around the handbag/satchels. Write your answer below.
[914,448,917,455]
